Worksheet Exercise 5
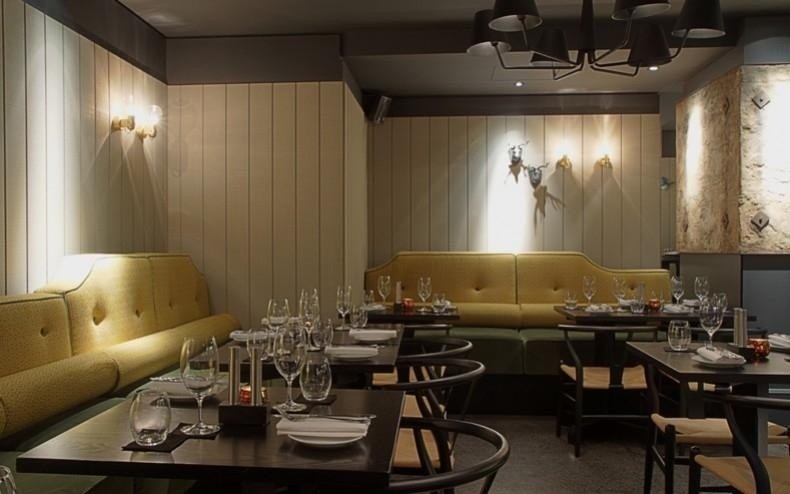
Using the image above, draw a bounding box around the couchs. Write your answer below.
[365,251,678,373]
[1,249,242,432]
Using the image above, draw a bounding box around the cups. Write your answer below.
[128,388,172,447]
[733,306,748,346]
[668,319,690,352]
[630,295,644,313]
[751,337,770,357]
[564,291,577,310]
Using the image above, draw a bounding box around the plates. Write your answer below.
[327,346,378,359]
[683,299,699,306]
[231,329,266,342]
[288,435,362,447]
[350,329,396,340]
[692,354,746,368]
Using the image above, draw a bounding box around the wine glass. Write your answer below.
[612,278,626,312]
[180,336,220,437]
[694,275,728,351]
[247,287,333,412]
[582,273,595,309]
[670,275,683,308]
[335,275,449,329]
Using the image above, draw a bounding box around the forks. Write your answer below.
[275,403,377,421]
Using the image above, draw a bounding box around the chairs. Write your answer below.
[557,326,660,458]
[692,389,790,494]
[373,359,483,471]
[390,336,470,380]
[383,418,506,493]
[641,364,780,493]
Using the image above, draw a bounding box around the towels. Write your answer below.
[696,347,744,363]
[276,417,371,438]
[349,328,397,337]
[325,344,378,355]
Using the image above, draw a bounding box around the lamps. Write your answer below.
[468,0,726,80]
